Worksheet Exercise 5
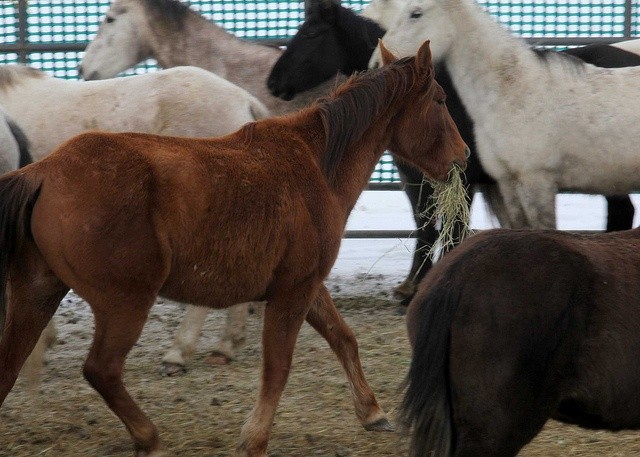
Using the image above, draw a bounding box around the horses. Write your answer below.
[265,1,640,315]
[77,0,350,127]
[390,227,640,457]
[0,36,470,457]
[1,63,282,379]
